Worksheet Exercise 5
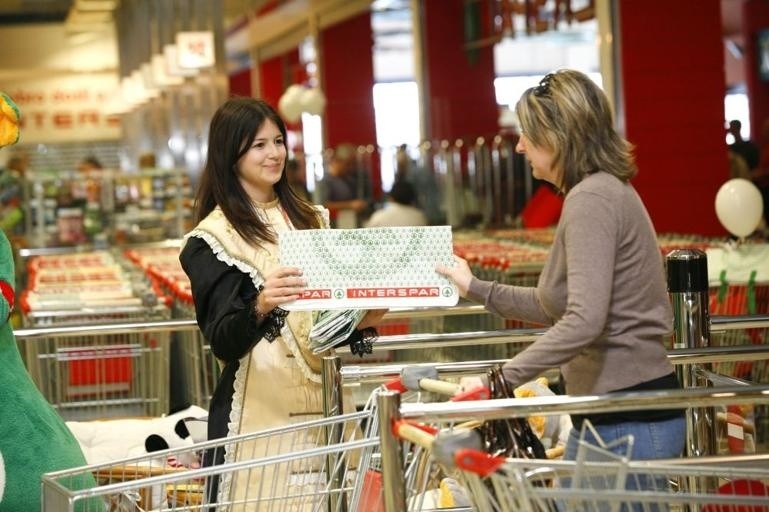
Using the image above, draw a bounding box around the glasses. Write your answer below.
[534,73,557,99]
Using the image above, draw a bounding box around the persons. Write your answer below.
[281,128,569,234]
[2,154,40,240]
[173,94,394,512]
[54,155,108,219]
[430,67,689,512]
[723,119,758,177]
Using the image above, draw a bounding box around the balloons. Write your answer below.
[711,175,765,241]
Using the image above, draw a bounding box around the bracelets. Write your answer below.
[252,305,271,320]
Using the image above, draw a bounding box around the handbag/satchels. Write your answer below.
[478,363,552,500]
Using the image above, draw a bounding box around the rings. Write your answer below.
[461,386,465,393]
